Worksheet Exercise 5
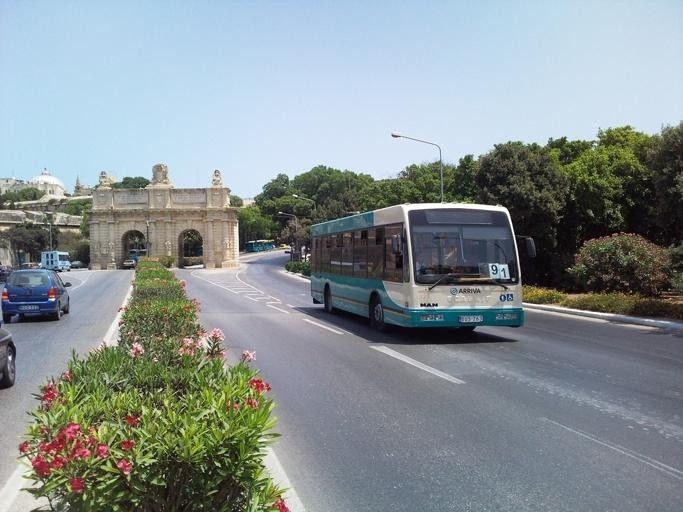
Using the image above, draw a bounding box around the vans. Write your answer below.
[41,251,71,272]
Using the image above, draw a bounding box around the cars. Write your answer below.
[0,322,16,389]
[123,260,136,270]
[0,265,13,282]
[2,268,72,323]
[280,244,289,249]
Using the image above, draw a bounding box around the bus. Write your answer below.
[129,250,146,260]
[309,202,536,332]
[246,240,274,253]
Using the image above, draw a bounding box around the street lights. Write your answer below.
[392,133,443,202]
[279,212,297,232]
[292,194,316,211]
